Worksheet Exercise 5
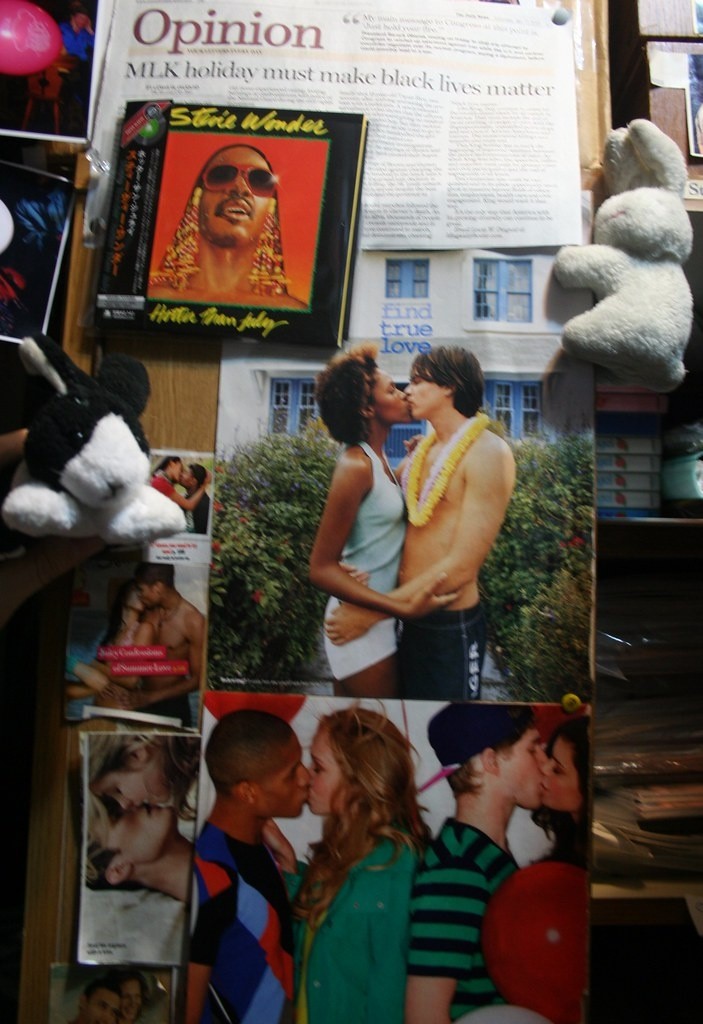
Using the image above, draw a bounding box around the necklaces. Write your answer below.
[400,409,490,526]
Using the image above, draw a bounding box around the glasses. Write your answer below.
[200,161,280,198]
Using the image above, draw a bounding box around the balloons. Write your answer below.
[453,861,590,1023]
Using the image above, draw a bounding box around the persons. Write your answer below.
[324,346,516,701]
[86,807,191,901]
[309,345,464,699]
[293,707,434,1024]
[187,709,313,1024]
[147,143,308,311]
[531,715,588,865]
[85,733,201,809]
[403,702,550,1023]
[178,463,210,533]
[148,456,210,509]
[67,563,205,725]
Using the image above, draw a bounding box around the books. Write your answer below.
[594,393,703,517]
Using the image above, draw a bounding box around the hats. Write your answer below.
[416,703,535,792]
[190,463,208,486]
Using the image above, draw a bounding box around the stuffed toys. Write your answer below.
[553,117,692,391]
